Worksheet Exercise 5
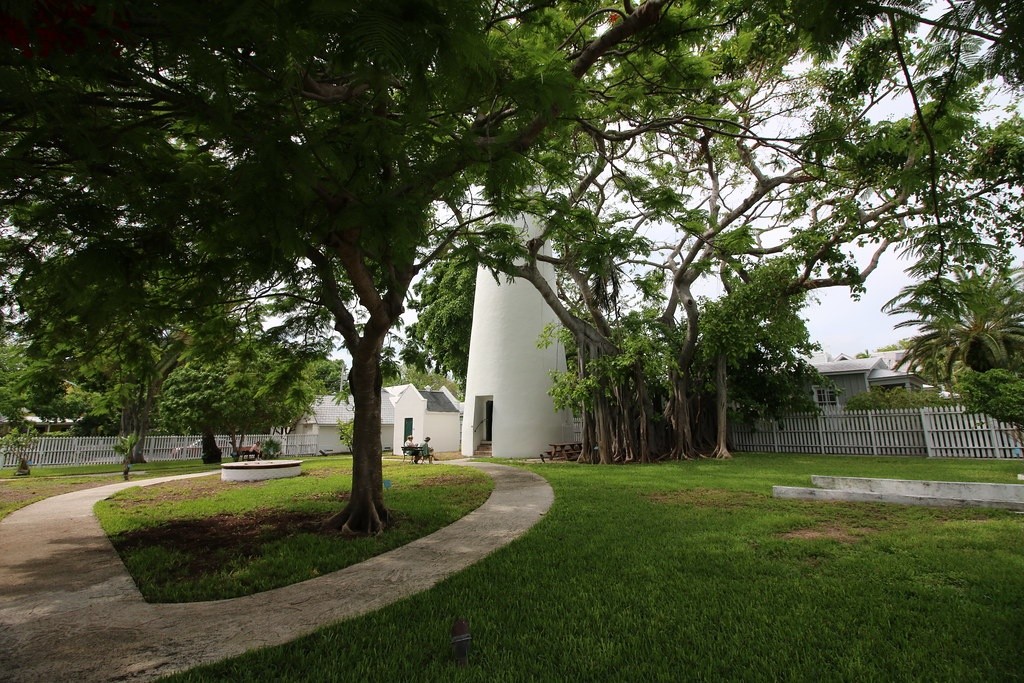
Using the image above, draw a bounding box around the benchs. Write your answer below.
[538,449,581,461]
[241,450,257,460]
[400,446,432,465]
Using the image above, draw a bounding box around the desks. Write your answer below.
[548,442,582,459]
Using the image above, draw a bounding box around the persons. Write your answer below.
[248,442,260,460]
[418,437,440,464]
[405,435,423,464]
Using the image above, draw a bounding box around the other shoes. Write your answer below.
[259,458,262,460]
[255,458,258,461]
[414,460,418,464]
[435,458,440,460]
